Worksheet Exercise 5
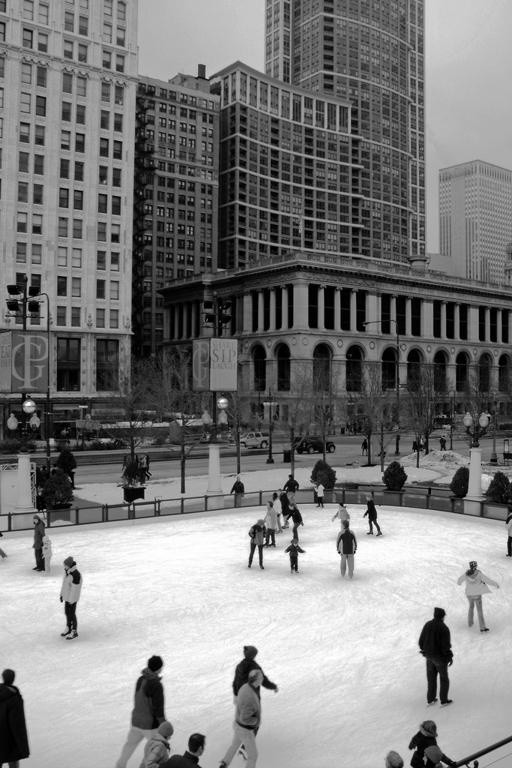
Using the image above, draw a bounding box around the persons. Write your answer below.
[230,477,244,507]
[386,720,454,768]
[505,512,512,557]
[361,439,367,456]
[139,454,153,482]
[60,556,80,640]
[248,474,382,579]
[0,669,31,768]
[32,515,45,571]
[418,607,454,704]
[457,561,499,631]
[440,436,446,450]
[42,536,52,574]
[116,645,279,768]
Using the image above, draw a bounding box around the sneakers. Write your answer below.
[61,628,70,636]
[66,630,78,640]
[427,698,437,703]
[376,531,383,536]
[441,699,452,704]
[367,531,373,534]
[37,568,45,571]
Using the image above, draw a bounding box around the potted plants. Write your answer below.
[481,471,512,521]
[309,460,337,503]
[382,461,408,505]
[449,467,469,514]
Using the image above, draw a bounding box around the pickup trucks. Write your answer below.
[233,432,270,449]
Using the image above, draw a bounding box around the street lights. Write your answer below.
[262,386,278,464]
[5,274,56,512]
[463,411,492,502]
[360,319,401,453]
[193,290,238,497]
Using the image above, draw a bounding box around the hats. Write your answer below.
[386,750,403,768]
[63,556,73,567]
[424,744,444,764]
[243,645,257,662]
[158,721,173,737]
[148,656,163,671]
[433,607,445,619]
[33,515,39,519]
[419,720,438,738]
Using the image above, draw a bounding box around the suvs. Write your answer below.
[294,436,335,454]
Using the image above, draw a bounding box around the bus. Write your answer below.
[433,413,496,432]
[90,418,206,447]
